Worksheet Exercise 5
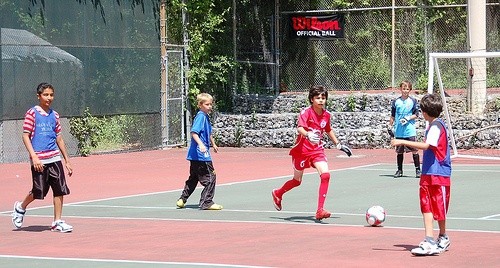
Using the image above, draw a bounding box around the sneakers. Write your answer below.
[50,219,73,233]
[13,201,26,229]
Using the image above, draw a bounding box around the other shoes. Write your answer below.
[271,189,282,211]
[176,199,185,207]
[436,235,450,252]
[316,210,331,219]
[394,170,403,177]
[410,239,440,255]
[209,204,223,209]
[416,170,421,177]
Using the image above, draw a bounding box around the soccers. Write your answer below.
[366,205,385,226]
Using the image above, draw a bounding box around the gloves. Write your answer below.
[336,143,352,157]
[306,130,320,144]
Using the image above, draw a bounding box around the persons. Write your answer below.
[12,83,73,232]
[177,93,223,210]
[390,93,452,256]
[272,85,352,221]
[390,81,422,177]
